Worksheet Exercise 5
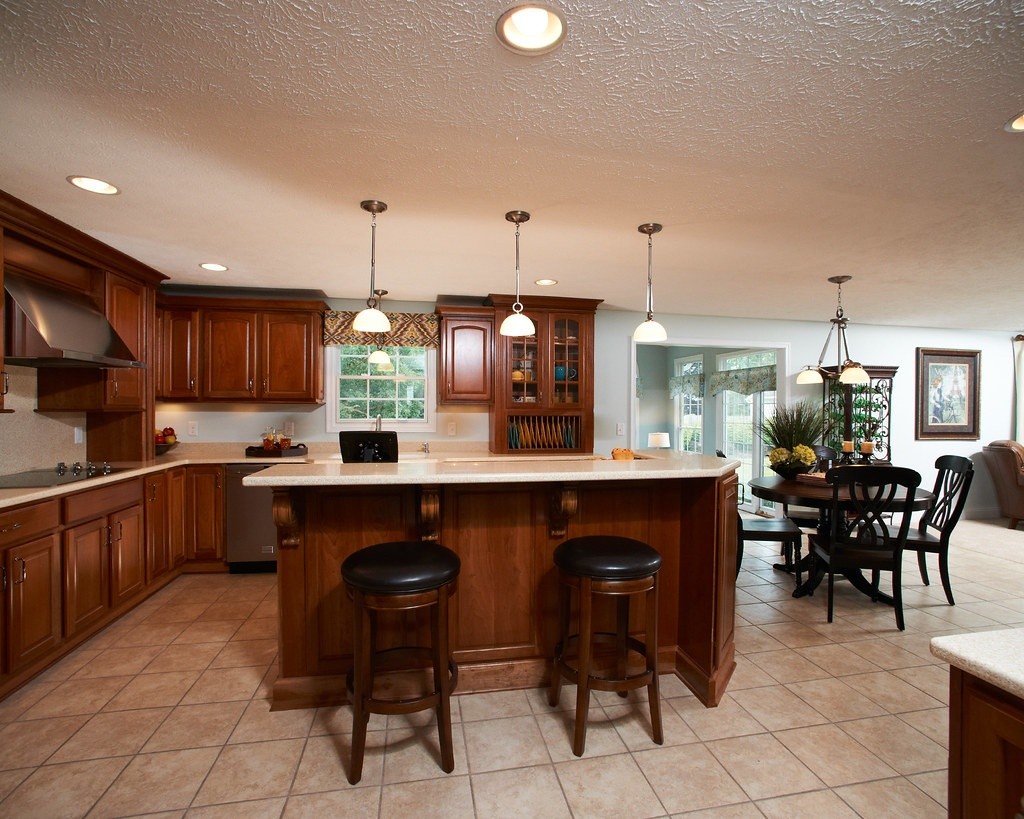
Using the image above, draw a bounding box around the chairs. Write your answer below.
[715,445,975,632]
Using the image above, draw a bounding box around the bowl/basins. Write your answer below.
[155,441,181,455]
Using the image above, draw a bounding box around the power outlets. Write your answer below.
[448,423,456,435]
[189,422,198,436]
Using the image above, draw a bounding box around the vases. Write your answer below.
[770,466,814,480]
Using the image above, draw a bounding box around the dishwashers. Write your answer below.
[226,464,278,575]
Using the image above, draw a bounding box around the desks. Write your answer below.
[749,476,935,606]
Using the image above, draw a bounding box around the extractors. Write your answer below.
[3,271,146,371]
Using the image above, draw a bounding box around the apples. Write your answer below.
[154,427,177,445]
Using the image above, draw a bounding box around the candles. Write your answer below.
[843,441,854,451]
[862,442,873,453]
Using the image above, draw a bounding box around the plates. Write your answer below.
[796,473,833,488]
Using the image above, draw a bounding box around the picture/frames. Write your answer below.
[914,347,982,441]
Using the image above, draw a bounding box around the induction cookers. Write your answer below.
[0,462,135,489]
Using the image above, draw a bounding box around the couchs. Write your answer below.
[982,440,1024,529]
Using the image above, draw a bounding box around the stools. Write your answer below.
[548,535,663,757]
[341,541,461,785]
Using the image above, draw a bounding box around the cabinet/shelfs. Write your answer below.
[434,294,605,453]
[929,628,1024,819]
[820,365,899,462]
[0,463,225,703]
[0,189,331,413]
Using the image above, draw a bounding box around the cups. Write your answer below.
[556,366,576,380]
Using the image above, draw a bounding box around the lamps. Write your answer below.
[796,275,871,384]
[377,362,394,372]
[648,432,670,450]
[500,210,536,336]
[368,289,390,364]
[353,200,390,332]
[633,223,668,341]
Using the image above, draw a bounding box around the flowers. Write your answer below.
[758,398,831,470]
